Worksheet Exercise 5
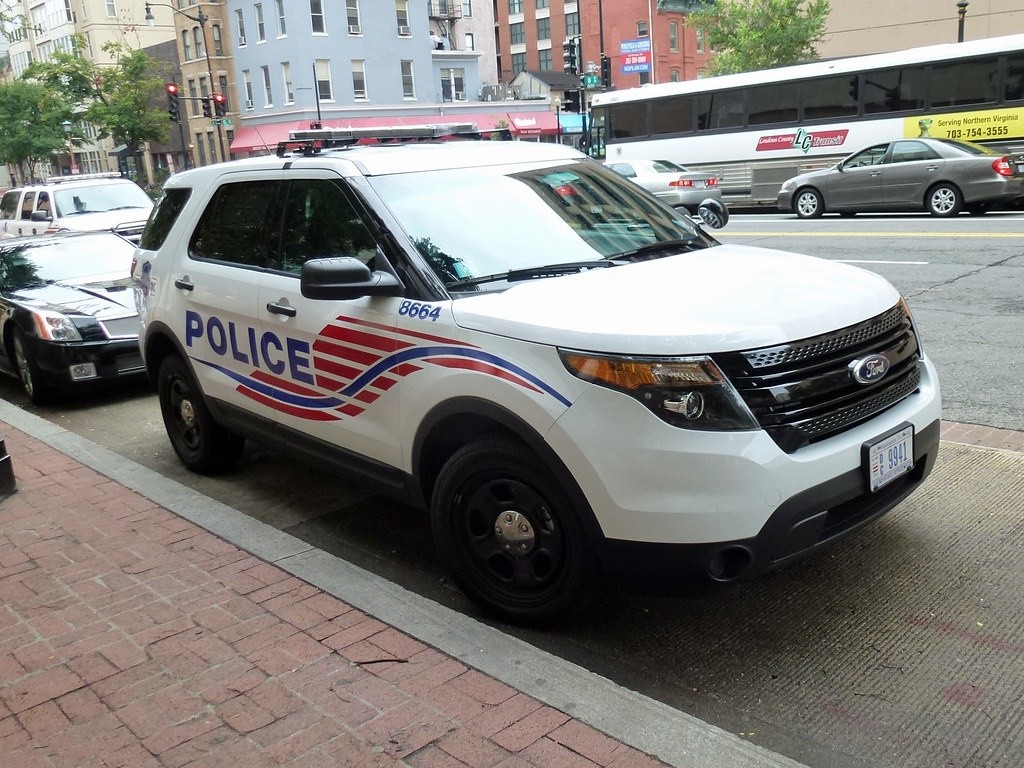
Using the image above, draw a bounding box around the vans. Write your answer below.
[0,170,157,245]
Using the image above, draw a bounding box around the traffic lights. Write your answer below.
[564,86,584,114]
[166,83,180,124]
[201,96,212,118]
[562,38,576,76]
[213,91,226,116]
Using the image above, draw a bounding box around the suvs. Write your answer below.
[131,122,942,631]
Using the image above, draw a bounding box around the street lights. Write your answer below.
[144,0,227,163]
[61,118,78,174]
[954,0,970,41]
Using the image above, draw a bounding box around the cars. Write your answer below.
[0,231,148,411]
[778,137,1024,219]
[599,157,720,213]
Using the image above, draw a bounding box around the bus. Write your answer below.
[580,33,1024,208]
